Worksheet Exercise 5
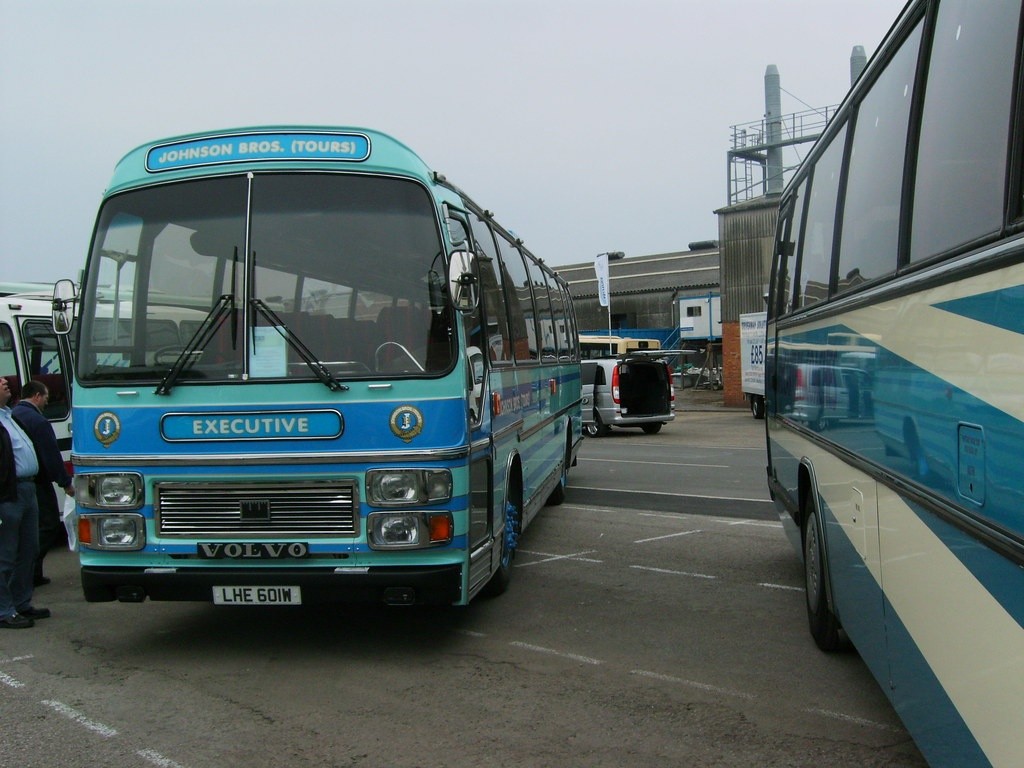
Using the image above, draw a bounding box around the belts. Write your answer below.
[17,476,36,482]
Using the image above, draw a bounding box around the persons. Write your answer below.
[12,383,75,584]
[0,378,53,628]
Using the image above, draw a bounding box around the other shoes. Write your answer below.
[42,577,50,584]
[19,607,50,619]
[0,612,35,628]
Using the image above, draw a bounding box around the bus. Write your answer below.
[757,1,1023,768]
[50,122,586,610]
[579,334,661,361]
[0,294,214,535]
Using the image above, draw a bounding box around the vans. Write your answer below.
[581,347,706,437]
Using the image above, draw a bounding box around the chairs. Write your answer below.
[376,307,425,357]
[284,311,310,376]
[419,309,434,338]
[309,314,336,376]
[356,320,375,359]
[334,318,357,371]
[217,310,285,368]
